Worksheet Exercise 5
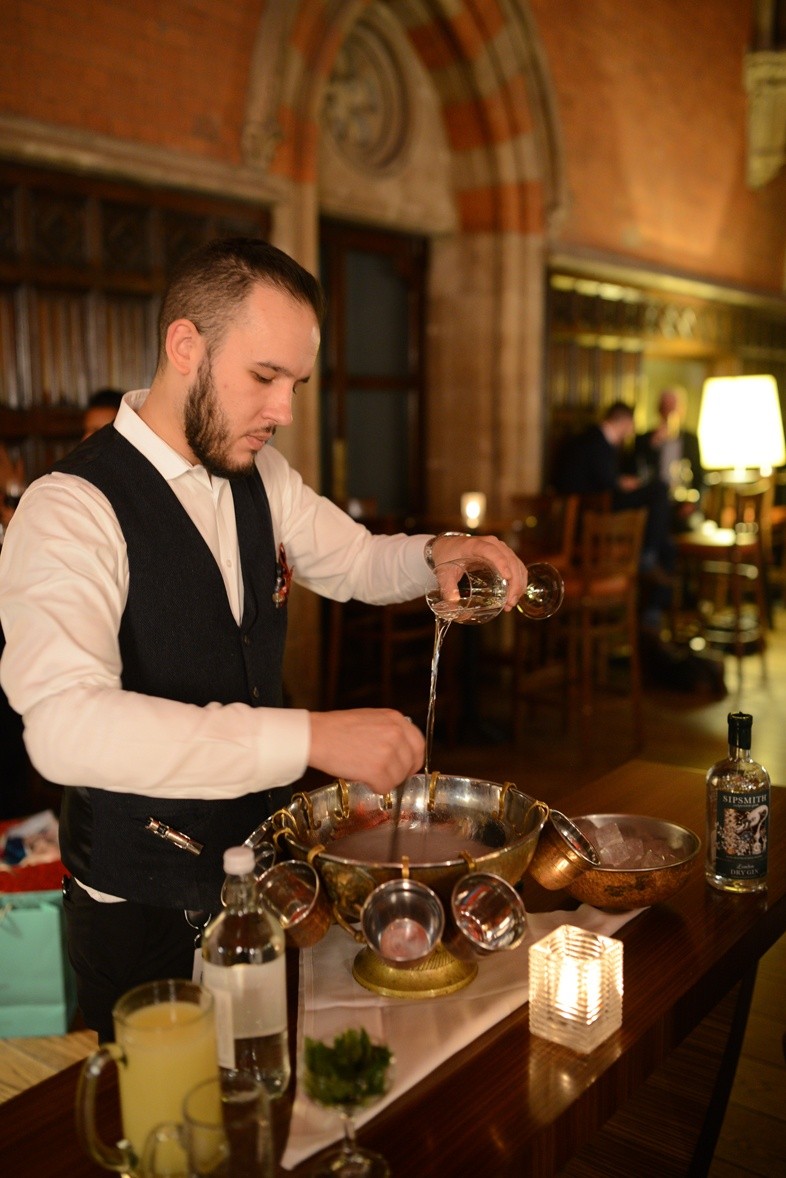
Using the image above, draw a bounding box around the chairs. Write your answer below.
[342,473,786,754]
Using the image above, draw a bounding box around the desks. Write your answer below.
[665,524,770,703]
[1,762,786,1178]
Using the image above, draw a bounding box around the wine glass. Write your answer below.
[302,1069,390,1178]
[425,559,564,624]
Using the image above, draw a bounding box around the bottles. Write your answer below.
[707,711,771,893]
[203,844,289,1105]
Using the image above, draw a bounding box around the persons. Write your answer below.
[0,236,529,1045]
[557,391,703,536]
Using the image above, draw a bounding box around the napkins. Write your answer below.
[279,905,652,1172]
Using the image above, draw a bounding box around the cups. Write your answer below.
[527,808,599,892]
[255,860,332,949]
[360,878,444,970]
[128,1073,275,1178]
[77,979,230,1178]
[528,924,624,1055]
[441,874,527,964]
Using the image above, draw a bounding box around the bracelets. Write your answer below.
[426,532,471,570]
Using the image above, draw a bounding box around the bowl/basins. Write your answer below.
[282,773,545,919]
[567,815,701,915]
